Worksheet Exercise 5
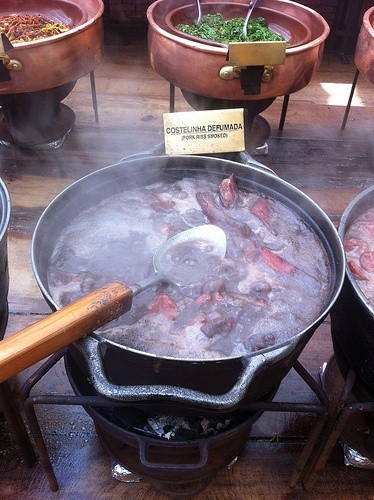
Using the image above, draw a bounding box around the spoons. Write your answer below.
[1,223,230,390]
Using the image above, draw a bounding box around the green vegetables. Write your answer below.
[175,12,290,46]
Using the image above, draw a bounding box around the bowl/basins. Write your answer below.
[145,0,332,100]
[353,6,374,83]
[1,0,106,96]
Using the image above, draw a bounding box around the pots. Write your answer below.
[336,183,374,314]
[0,179,11,339]
[30,154,346,403]
